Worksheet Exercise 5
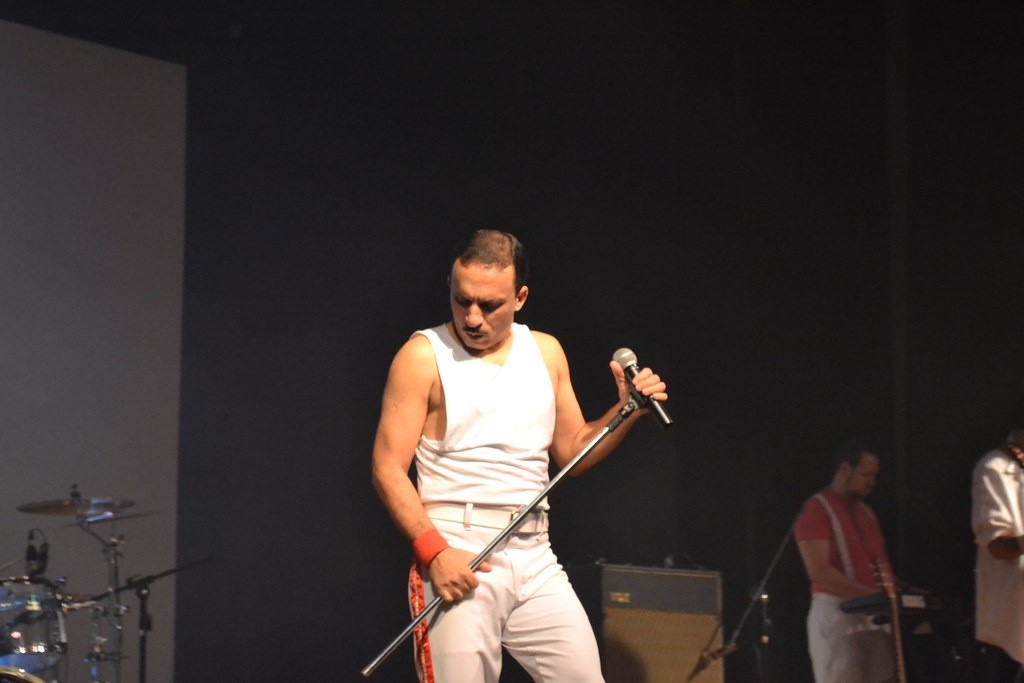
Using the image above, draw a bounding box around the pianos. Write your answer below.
[840,591,972,682]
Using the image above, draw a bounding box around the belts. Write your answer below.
[425,502,550,535]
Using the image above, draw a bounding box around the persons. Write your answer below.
[368,227,669,683]
[794,440,907,683]
[971,412,1024,683]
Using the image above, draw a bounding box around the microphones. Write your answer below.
[612,347,674,431]
[24,530,40,574]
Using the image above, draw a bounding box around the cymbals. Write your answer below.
[18,497,134,516]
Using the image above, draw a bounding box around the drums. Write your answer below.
[0,575,67,674]
